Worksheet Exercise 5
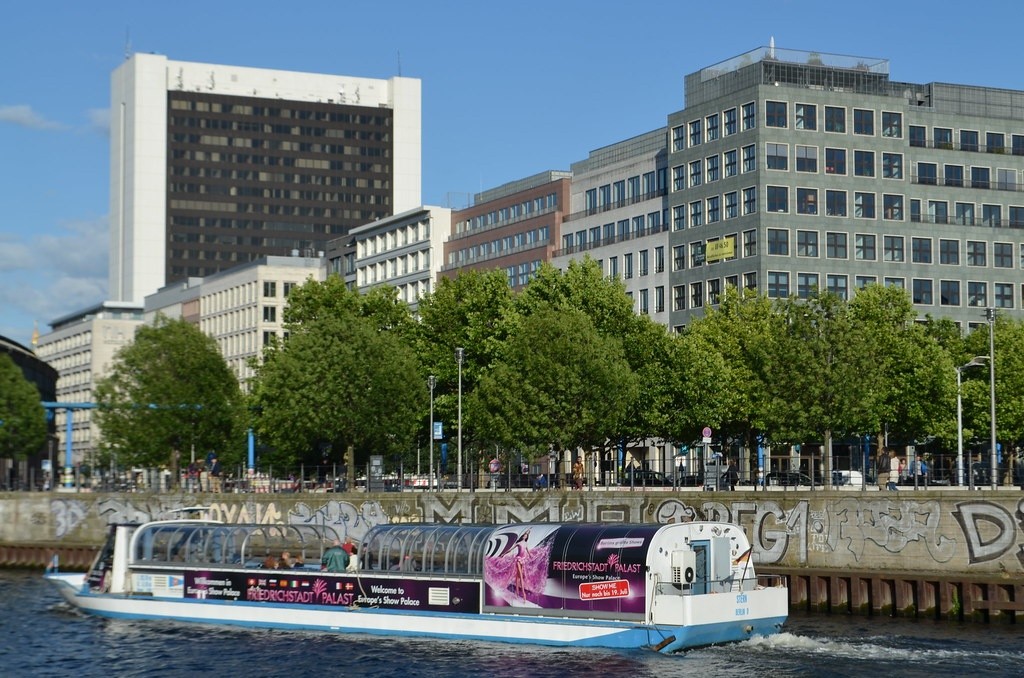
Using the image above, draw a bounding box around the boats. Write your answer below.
[42,519,789,652]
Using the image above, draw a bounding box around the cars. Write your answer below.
[617,462,1006,485]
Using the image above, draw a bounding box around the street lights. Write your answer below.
[981,307,1000,491]
[454,347,464,492]
[426,376,435,492]
[957,356,991,485]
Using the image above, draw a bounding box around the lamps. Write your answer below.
[672,550,698,585]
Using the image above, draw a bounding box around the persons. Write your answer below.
[186,459,205,494]
[996,446,1024,490]
[287,471,296,482]
[572,456,584,490]
[391,554,420,572]
[262,551,304,571]
[209,458,223,493]
[721,458,740,491]
[323,536,375,575]
[534,474,547,488]
[876,447,928,491]
